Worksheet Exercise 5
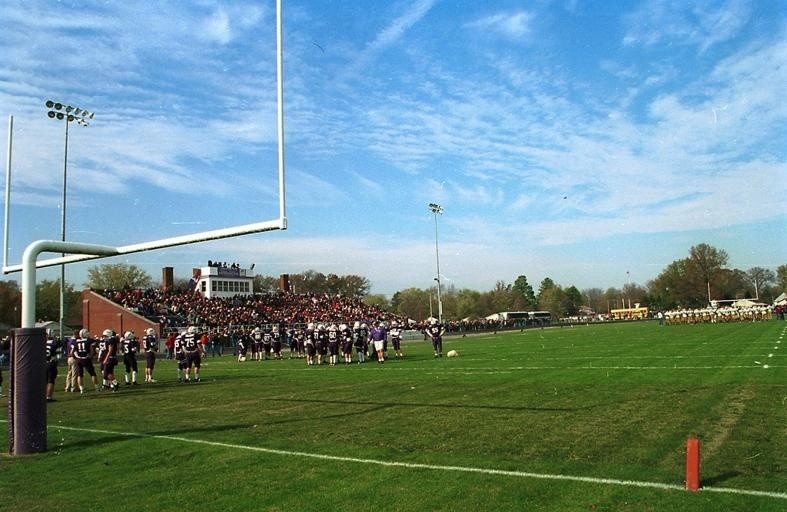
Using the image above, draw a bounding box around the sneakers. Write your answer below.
[308,355,387,366]
[65,380,121,395]
[46,398,57,402]
[178,377,201,384]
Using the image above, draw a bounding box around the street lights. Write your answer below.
[13,306,18,328]
[625,269,633,307]
[432,277,442,321]
[428,201,445,277]
[42,100,95,341]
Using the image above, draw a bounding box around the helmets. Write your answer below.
[430,318,439,325]
[102,329,113,340]
[188,326,197,334]
[307,321,397,333]
[124,332,135,340]
[79,329,89,339]
[45,328,55,338]
[146,328,156,338]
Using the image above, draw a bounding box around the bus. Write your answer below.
[498,310,529,324]
[526,310,554,321]
[610,307,648,321]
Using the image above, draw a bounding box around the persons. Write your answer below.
[43,283,446,407]
[0,354,7,398]
[607,310,652,320]
[445,316,550,332]
[654,305,787,325]
